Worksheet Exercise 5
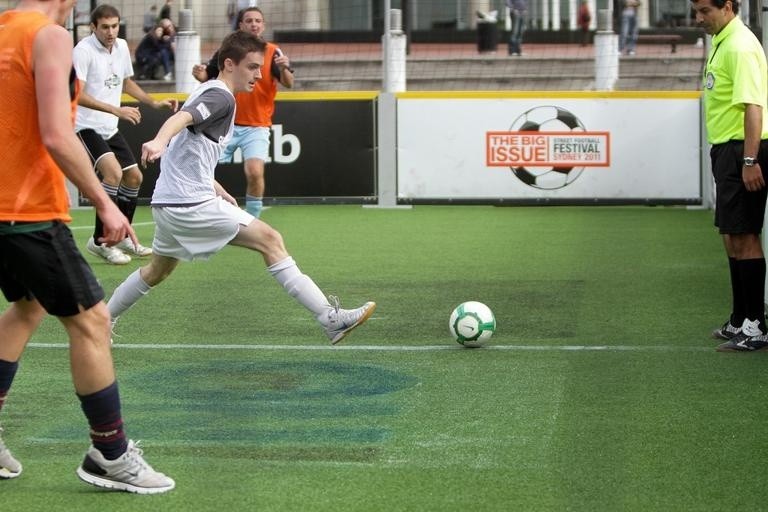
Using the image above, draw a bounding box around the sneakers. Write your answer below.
[714,317,768,353]
[615,49,637,57]
[0,427,23,478]
[137,71,172,83]
[115,238,154,256]
[510,51,527,57]
[78,443,175,494]
[86,237,133,264]
[322,300,376,344]
[693,39,704,49]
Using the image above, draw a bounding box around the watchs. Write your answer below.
[742,157,757,168]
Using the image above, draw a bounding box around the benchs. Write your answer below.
[626,34,681,53]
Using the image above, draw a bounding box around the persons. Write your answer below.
[614,0,642,56]
[578,0,591,46]
[691,1,768,352]
[506,1,528,55]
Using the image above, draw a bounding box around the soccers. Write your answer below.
[449,301,496,348]
[506,105,589,191]
